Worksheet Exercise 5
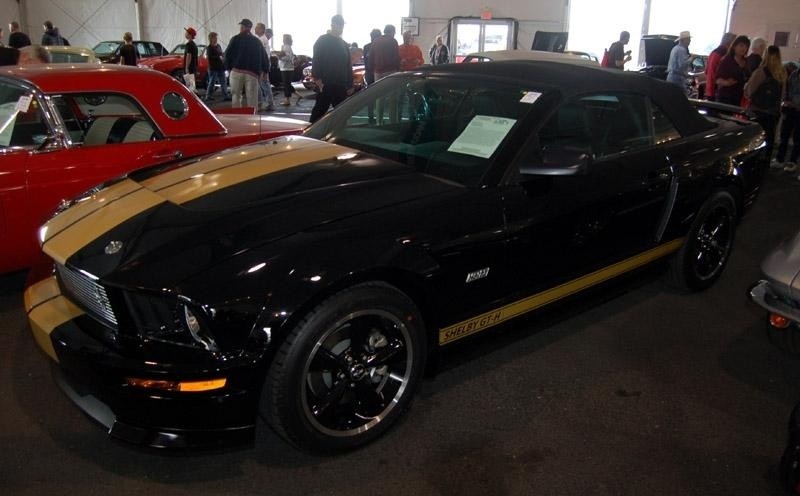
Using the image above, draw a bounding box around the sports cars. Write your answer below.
[19,57,774,462]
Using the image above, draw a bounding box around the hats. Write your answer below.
[184,26,196,38]
[673,31,692,44]
[239,20,252,28]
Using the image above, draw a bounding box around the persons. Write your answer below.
[348,42,363,64]
[363,24,424,125]
[40,20,71,46]
[8,21,31,49]
[307,13,355,124]
[105,31,141,67]
[223,19,270,113]
[609,30,633,71]
[429,34,448,66]
[666,31,800,172]
[255,22,277,110]
[182,27,198,87]
[272,34,304,104]
[205,31,233,102]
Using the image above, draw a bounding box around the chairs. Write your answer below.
[432,92,666,162]
[79,117,155,147]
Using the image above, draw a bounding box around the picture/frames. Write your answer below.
[401,16,419,37]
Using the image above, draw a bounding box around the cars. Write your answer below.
[0,39,366,98]
[456,33,709,99]
[0,64,307,275]
[742,227,800,362]
